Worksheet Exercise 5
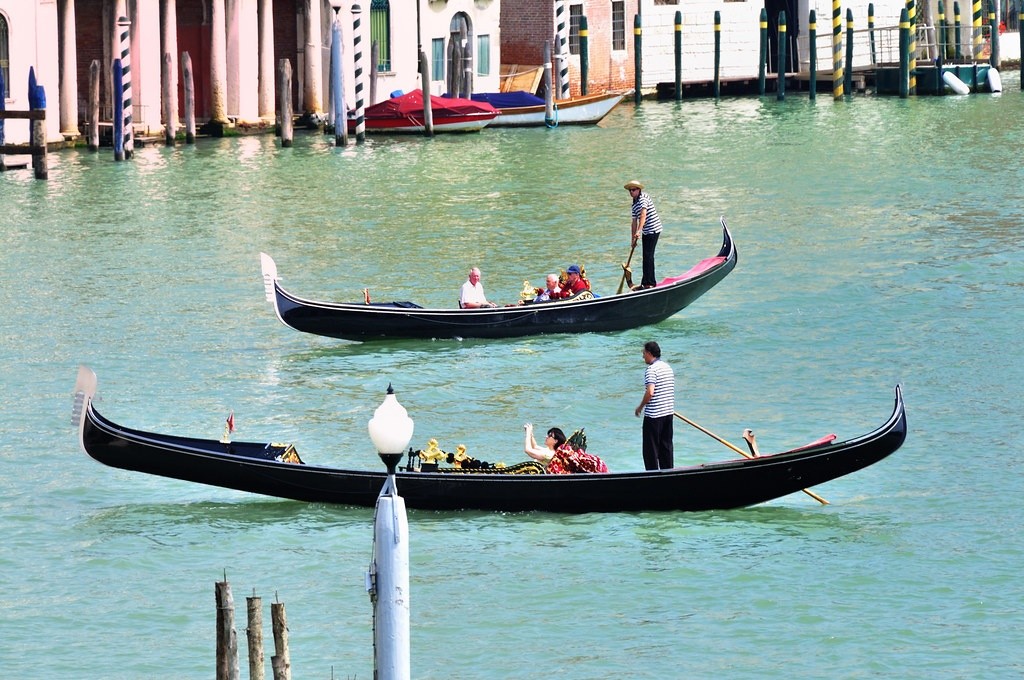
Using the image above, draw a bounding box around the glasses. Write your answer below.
[629,188,639,192]
[548,433,556,440]
[567,272,577,275]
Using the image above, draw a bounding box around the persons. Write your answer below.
[461,268,497,307]
[624,181,662,290]
[635,342,675,469]
[533,265,587,303]
[524,423,566,464]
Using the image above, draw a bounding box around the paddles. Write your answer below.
[674,411,830,505]
[615,236,637,294]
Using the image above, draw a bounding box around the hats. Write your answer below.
[566,265,581,272]
[624,181,645,189]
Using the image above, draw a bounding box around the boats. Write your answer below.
[874,62,994,96]
[345,89,503,133]
[438,89,634,127]
[71,365,908,514]
[260,213,738,342]
